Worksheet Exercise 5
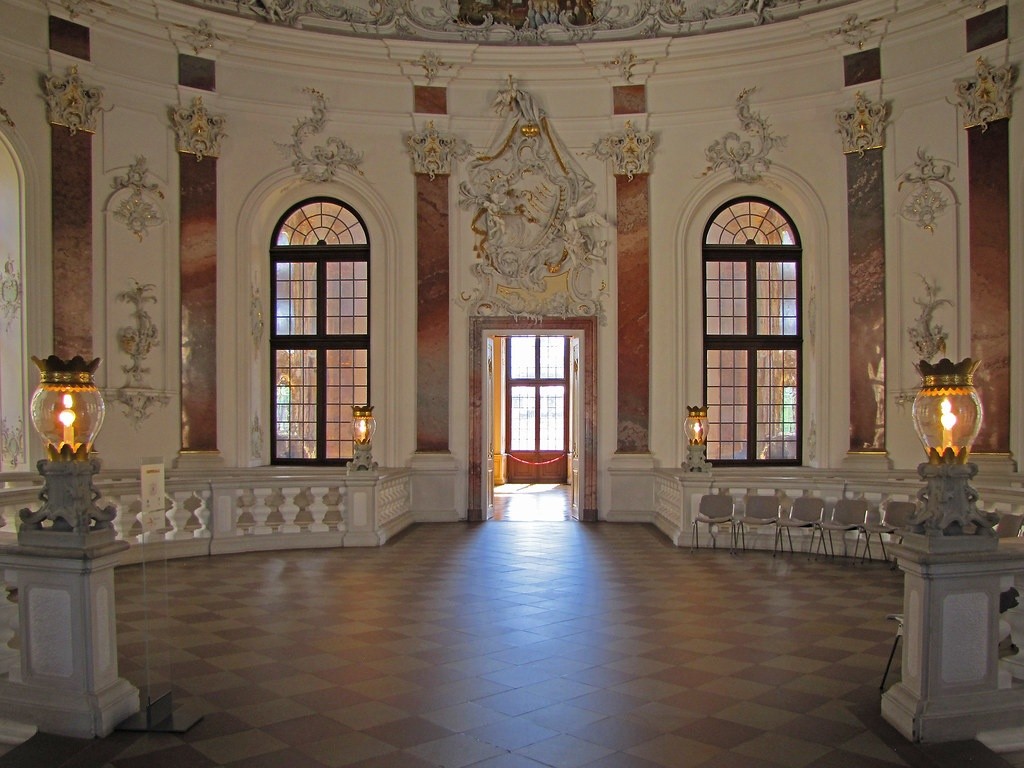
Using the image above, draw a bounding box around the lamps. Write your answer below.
[28,354,107,463]
[683,406,710,446]
[351,406,376,445]
[912,358,984,465]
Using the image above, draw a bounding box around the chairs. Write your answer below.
[691,495,737,554]
[735,496,783,558]
[816,500,872,564]
[773,497,827,561]
[879,612,903,691]
[861,502,917,565]
